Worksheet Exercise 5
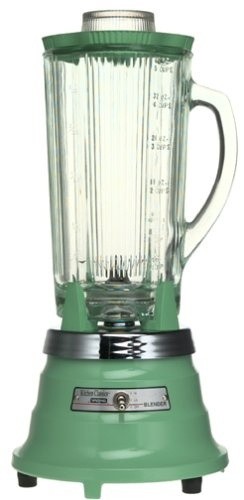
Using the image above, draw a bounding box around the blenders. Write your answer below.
[10,8,238,496]
